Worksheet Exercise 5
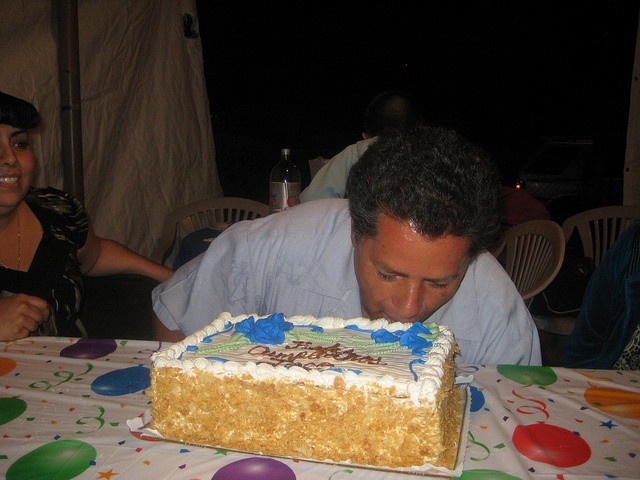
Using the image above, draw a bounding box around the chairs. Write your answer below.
[152,196,272,270]
[531,205,639,336]
[489,218,566,310]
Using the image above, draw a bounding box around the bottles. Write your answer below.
[269,148,301,211]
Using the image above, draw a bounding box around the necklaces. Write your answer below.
[1,206,22,269]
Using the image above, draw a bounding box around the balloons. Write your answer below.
[6,439,97,480]
[60,338,117,359]
[451,469,523,480]
[497,364,556,385]
[211,457,296,480]
[470,385,485,413]
[584,387,640,420]
[0,358,16,376]
[512,423,592,467]
[90,366,151,396]
[0,398,27,425]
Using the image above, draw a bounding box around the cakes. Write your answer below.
[150,311,468,472]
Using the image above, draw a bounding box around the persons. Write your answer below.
[152,122,542,367]
[484,142,550,237]
[0,89,176,342]
[293,88,413,207]
[550,218,640,370]
